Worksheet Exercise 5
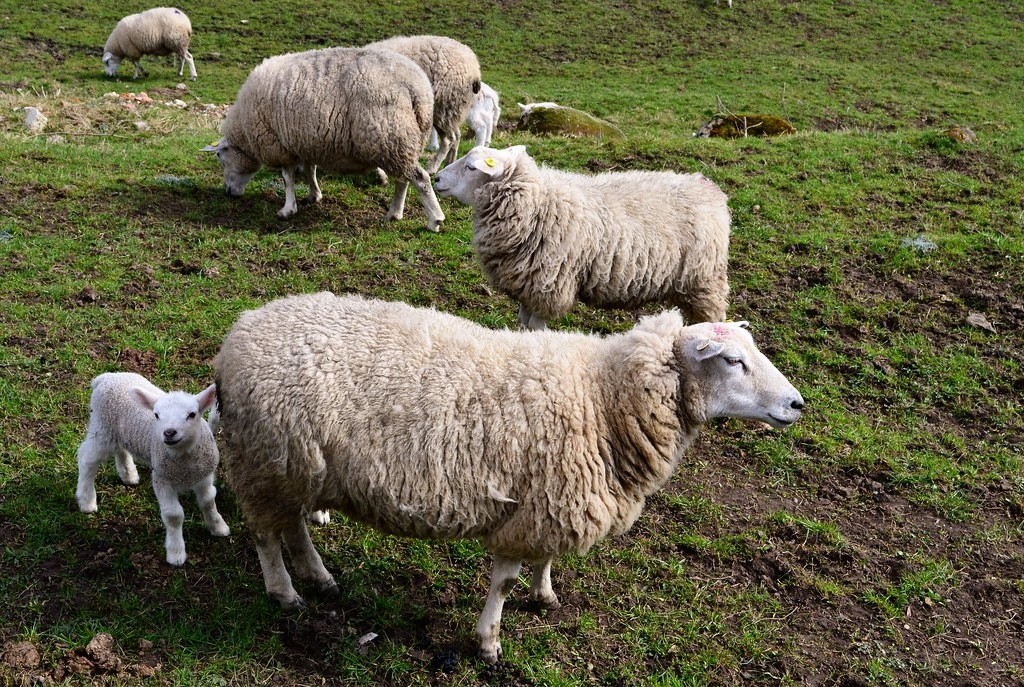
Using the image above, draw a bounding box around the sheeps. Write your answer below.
[73,369,232,566]
[101,7,198,80]
[435,145,732,331]
[205,292,805,666]
[197,35,501,235]
